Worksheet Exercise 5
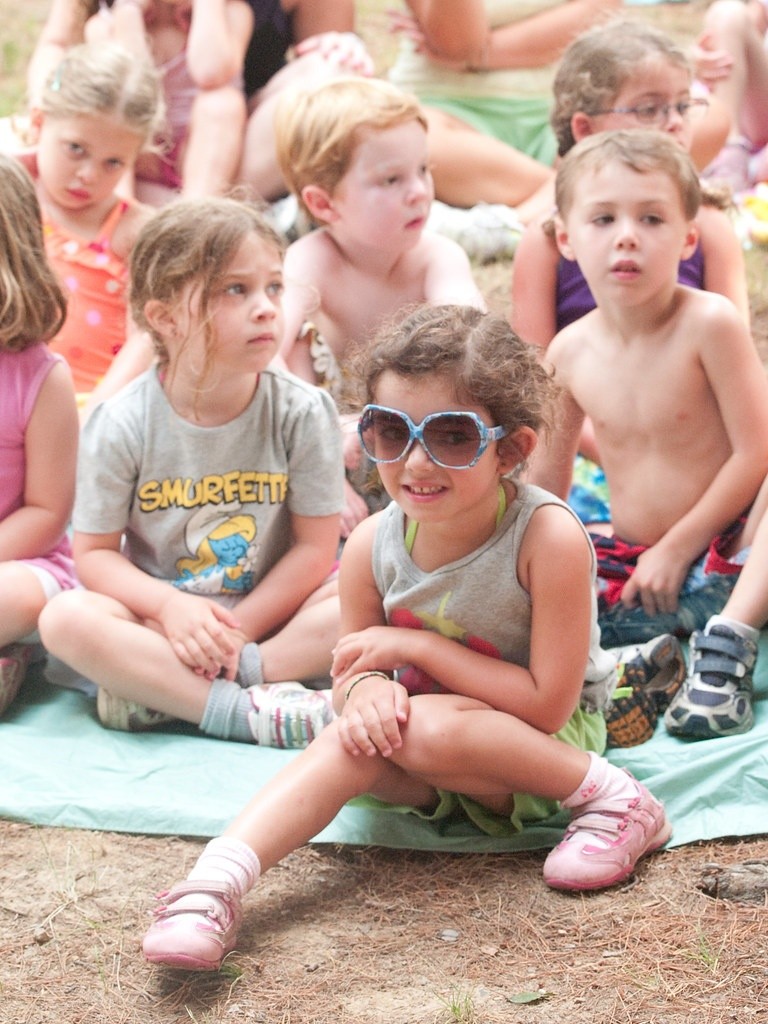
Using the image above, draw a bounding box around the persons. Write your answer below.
[37,195,342,750]
[144,306,674,973]
[20,0,768,262]
[0,153,79,717]
[527,130,768,748]
[274,78,486,553]
[1,45,165,411]
[511,19,749,536]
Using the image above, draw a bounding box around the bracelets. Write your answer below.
[346,671,389,699]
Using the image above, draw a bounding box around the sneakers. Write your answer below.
[246,679,341,750]
[666,623,758,736]
[97,686,181,729]
[602,632,686,746]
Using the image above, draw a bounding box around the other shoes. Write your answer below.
[143,880,242,969]
[0,643,36,712]
[544,766,671,890]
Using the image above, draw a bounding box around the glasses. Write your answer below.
[358,402,512,470]
[586,98,709,126]
